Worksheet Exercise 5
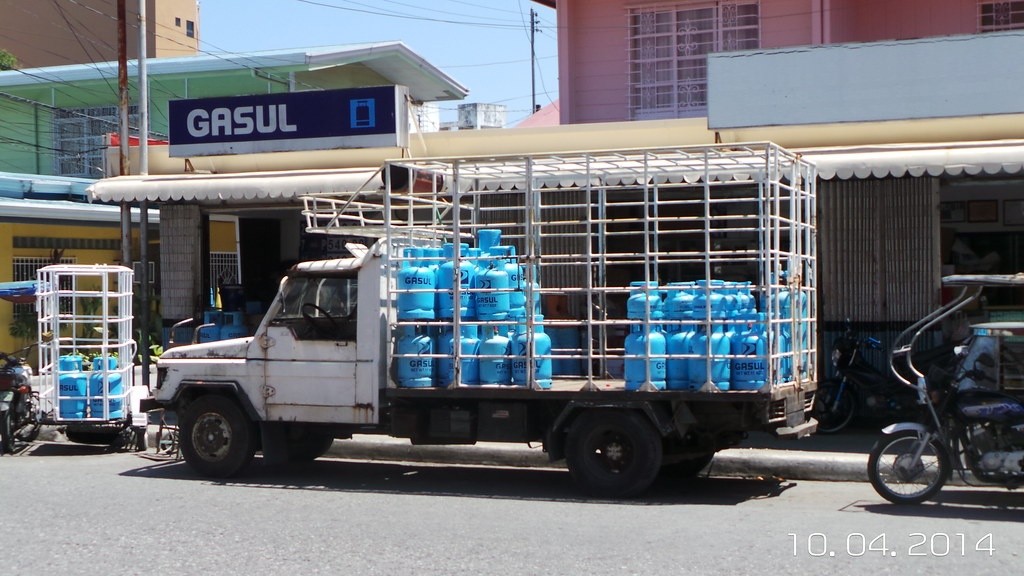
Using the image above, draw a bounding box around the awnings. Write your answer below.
[88,144,1021,202]
